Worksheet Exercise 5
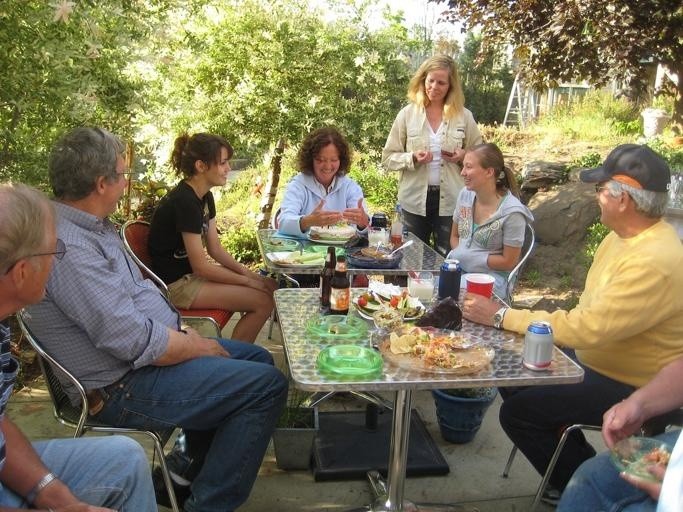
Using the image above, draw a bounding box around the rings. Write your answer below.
[353,217,357,221]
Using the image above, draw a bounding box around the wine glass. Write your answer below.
[370,309,402,346]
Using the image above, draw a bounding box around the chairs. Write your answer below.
[121,217,244,342]
[14,306,184,511]
[528,417,683,511]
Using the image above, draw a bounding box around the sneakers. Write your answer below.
[540,484,561,505]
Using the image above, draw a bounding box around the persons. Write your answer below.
[500,142,683,507]
[278,127,372,287]
[1,182,159,512]
[16,125,289,511]
[384,54,487,254]
[448,142,529,301]
[147,133,279,345]
[554,361,683,512]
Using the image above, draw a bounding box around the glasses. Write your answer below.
[116,167,138,182]
[593,182,610,192]
[3,236,69,276]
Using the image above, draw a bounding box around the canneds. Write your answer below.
[439,259,461,298]
[524,321,552,371]
[373,213,387,227]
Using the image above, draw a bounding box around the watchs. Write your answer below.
[493,307,506,329]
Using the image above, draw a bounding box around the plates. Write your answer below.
[353,292,426,323]
[304,314,368,339]
[265,252,323,268]
[261,236,299,251]
[378,326,497,376]
[306,231,345,244]
[316,345,385,377]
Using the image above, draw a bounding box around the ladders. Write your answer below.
[503,73,535,130]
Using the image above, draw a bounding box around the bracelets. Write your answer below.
[26,473,57,505]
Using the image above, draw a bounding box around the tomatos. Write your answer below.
[358,297,367,306]
[389,297,399,307]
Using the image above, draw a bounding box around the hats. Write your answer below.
[579,142,672,192]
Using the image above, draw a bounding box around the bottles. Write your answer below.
[318,244,349,315]
[367,204,404,249]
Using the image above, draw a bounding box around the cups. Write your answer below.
[407,269,434,302]
[465,273,494,298]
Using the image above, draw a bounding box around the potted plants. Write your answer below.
[269,354,321,469]
[430,377,497,445]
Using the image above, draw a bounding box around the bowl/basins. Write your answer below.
[344,246,405,266]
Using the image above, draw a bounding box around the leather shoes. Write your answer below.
[150,467,191,509]
